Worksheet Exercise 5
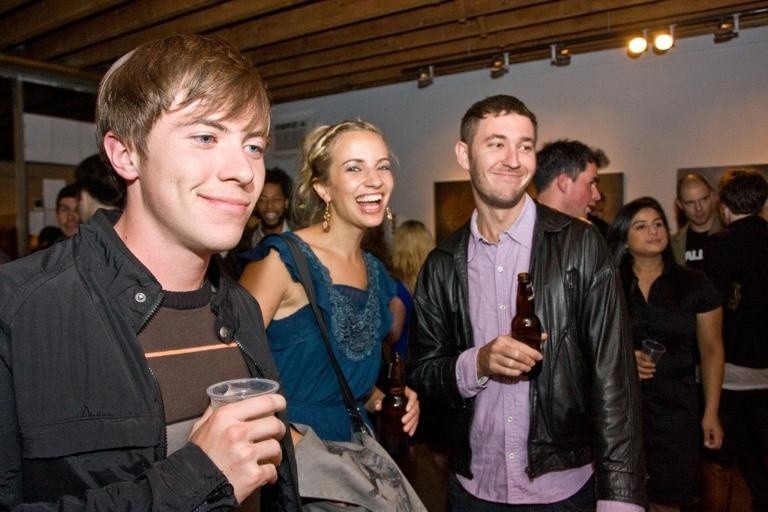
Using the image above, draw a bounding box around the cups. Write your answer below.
[639,338,666,363]
[208,375,280,418]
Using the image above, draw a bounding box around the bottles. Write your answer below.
[510,274,543,382]
[381,343,410,454]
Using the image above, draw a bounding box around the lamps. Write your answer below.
[714,16,740,44]
[547,44,572,67]
[419,65,435,87]
[489,53,511,81]
[627,28,675,56]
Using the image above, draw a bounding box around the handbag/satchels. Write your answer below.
[273,415,430,511]
